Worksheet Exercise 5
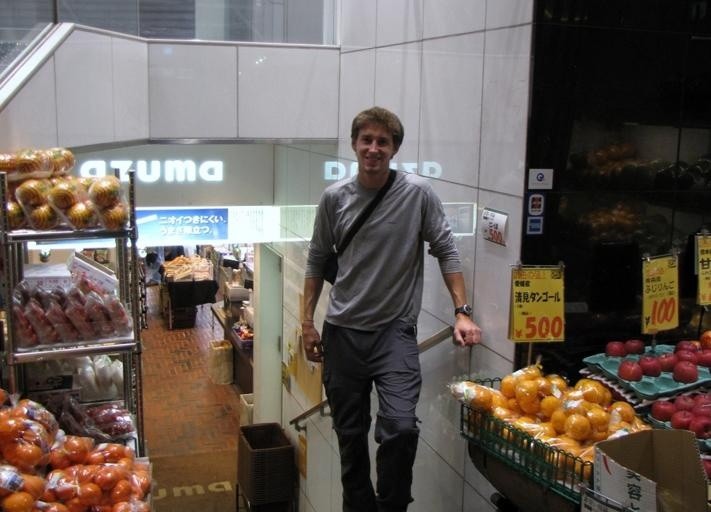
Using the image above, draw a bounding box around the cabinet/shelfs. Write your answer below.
[0,170,147,459]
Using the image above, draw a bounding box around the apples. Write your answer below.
[605,331,711,477]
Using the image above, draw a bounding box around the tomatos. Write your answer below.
[11,274,133,347]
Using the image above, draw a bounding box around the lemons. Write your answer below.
[452,364,652,478]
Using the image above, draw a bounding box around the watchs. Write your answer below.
[454,304,472,317]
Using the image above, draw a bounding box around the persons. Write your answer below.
[301,105,481,512]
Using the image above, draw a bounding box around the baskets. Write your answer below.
[234,422,297,505]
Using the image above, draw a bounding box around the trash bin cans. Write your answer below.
[238,423,294,511]
[209,340,234,385]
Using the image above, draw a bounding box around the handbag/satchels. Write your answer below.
[323,249,339,285]
